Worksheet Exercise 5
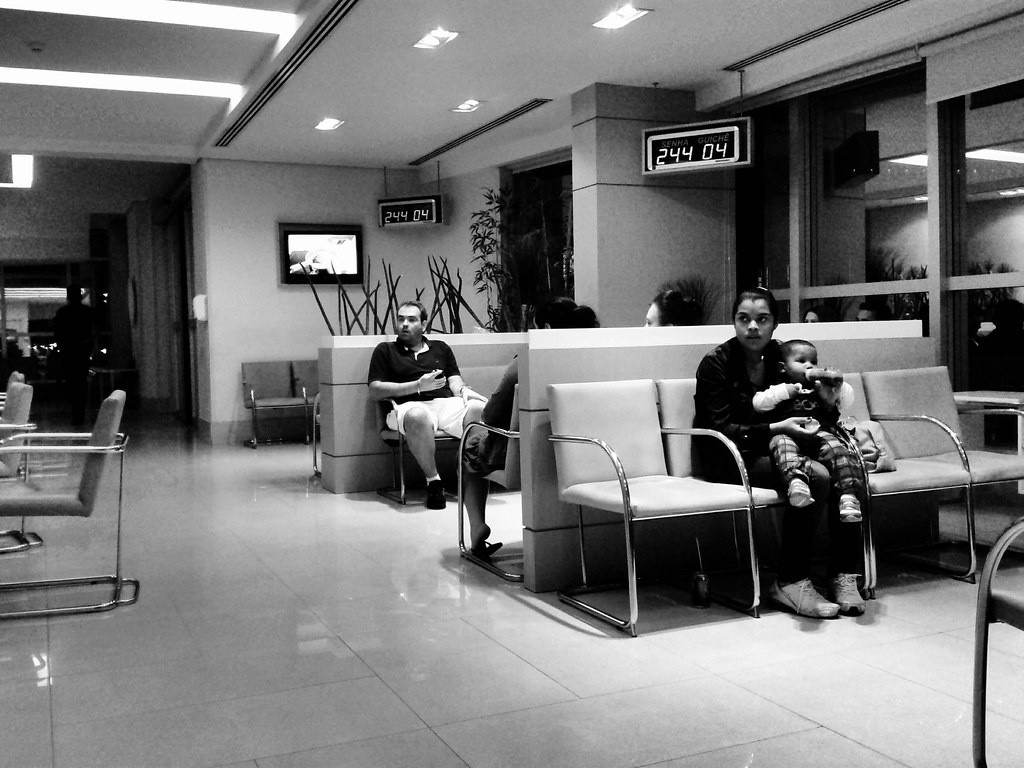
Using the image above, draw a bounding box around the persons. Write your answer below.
[367,300,489,510]
[462,297,600,560]
[643,275,721,327]
[855,300,892,321]
[751,337,863,523]
[802,306,828,323]
[689,289,867,619]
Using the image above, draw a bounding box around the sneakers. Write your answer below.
[768,578,840,618]
[839,498,863,522]
[787,482,815,509]
[834,573,866,617]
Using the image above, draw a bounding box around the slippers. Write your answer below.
[469,539,503,558]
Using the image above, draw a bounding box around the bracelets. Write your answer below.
[459,385,472,393]
[415,380,420,395]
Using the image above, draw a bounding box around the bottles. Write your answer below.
[806,369,844,388]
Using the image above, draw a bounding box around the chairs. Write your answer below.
[0,370,139,621]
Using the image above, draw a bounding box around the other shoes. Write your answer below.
[426,480,446,509]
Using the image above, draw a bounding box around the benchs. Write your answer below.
[548,366,1024,768]
[372,364,522,588]
[241,360,325,477]
